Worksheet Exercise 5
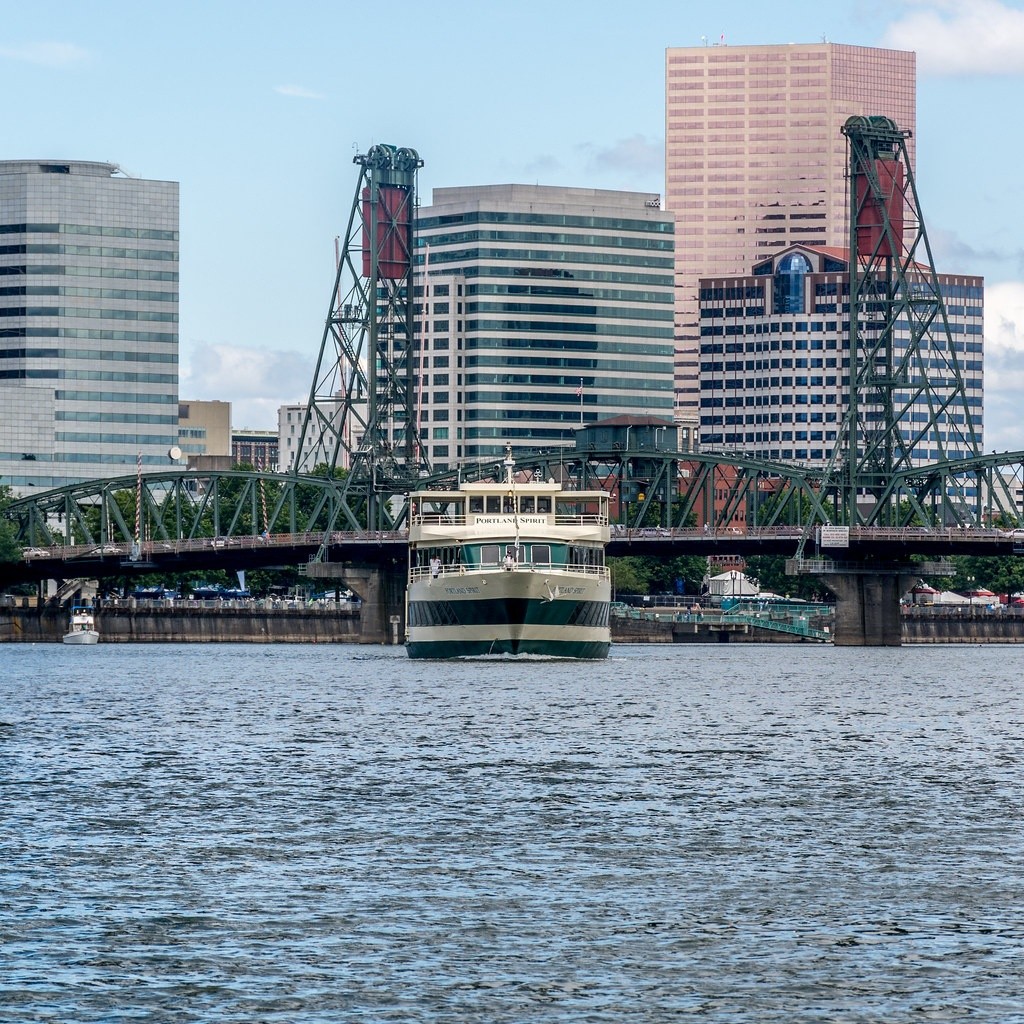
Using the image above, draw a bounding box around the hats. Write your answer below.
[507,550,511,554]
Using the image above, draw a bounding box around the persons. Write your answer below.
[686,602,704,621]
[472,504,483,513]
[703,524,709,536]
[75,610,80,617]
[504,502,514,512]
[503,550,515,571]
[261,529,269,544]
[429,554,441,578]
[656,524,660,534]
[80,609,87,616]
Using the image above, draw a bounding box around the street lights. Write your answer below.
[967,576,976,604]
[626,425,632,451]
[730,569,737,605]
[655,426,667,450]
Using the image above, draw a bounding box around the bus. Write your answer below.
[610,602,626,611]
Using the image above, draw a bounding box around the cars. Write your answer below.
[1004,528,1024,539]
[640,527,671,537]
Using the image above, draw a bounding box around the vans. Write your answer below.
[608,523,627,537]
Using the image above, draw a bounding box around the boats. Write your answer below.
[62,606,100,644]
[403,441,612,658]
